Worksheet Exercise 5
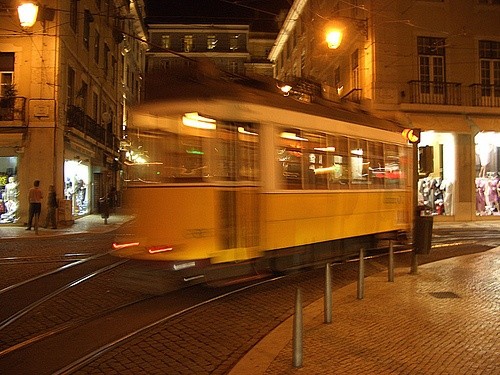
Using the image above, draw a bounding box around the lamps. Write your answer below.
[17,3,39,30]
[324,20,347,49]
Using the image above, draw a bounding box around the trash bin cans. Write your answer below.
[413,205,434,256]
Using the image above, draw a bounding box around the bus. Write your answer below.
[108,71,425,293]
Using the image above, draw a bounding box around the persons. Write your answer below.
[24,180,43,230]
[419,170,500,219]
[0,173,87,222]
[39,185,58,229]
